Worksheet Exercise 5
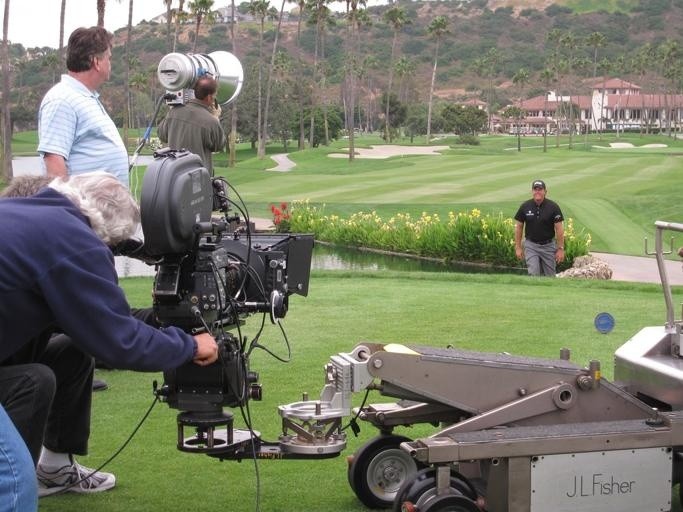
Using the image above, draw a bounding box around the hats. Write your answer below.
[532,180,546,190]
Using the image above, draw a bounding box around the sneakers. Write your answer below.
[36,454,117,498]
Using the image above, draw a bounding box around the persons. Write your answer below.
[156,74,227,178]
[514,180,565,277]
[0,175,159,499]
[36,26,130,391]
[0,168,218,512]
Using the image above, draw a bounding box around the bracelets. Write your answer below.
[559,247,564,249]
